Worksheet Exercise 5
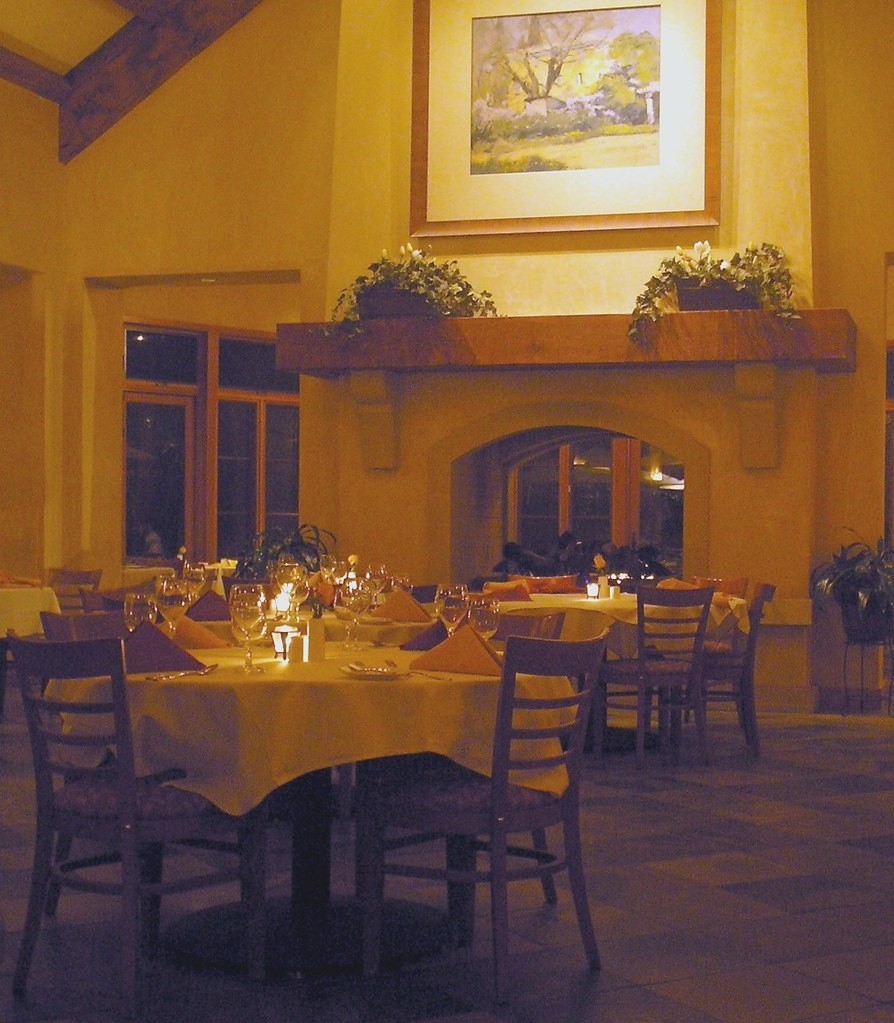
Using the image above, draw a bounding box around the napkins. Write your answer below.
[127,620,207,672]
[369,588,431,623]
[184,589,230,623]
[657,577,699,590]
[409,623,503,677]
[499,585,532,603]
[399,617,448,650]
[171,613,229,647]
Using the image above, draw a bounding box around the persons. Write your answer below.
[493,531,672,576]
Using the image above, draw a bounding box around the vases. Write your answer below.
[675,273,760,311]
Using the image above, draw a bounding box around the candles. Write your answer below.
[270,571,361,611]
[270,622,303,658]
[586,581,602,600]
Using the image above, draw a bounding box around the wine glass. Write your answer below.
[230,554,413,672]
[156,580,189,640]
[182,563,206,602]
[435,583,468,636]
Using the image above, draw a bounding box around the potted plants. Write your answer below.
[808,523,894,642]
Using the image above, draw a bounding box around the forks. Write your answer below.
[385,659,452,681]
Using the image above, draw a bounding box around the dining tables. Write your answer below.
[0,580,62,638]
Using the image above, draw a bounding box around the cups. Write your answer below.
[469,599,498,642]
[123,593,157,632]
[610,586,620,598]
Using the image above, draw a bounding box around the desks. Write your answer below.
[838,638,894,718]
[161,606,436,646]
[489,593,755,757]
[41,638,579,989]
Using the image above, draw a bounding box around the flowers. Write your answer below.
[306,238,508,342]
[622,240,805,341]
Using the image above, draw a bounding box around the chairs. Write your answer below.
[343,626,612,1010]
[589,580,719,769]
[509,573,578,594]
[664,582,777,759]
[4,625,275,1021]
[681,575,751,727]
[76,576,158,612]
[487,608,572,640]
[40,606,143,643]
[45,567,103,606]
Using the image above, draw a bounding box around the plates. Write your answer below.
[341,666,396,680]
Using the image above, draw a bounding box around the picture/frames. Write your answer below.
[407,0,726,241]
[357,283,440,319]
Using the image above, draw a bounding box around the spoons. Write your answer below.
[146,663,219,682]
